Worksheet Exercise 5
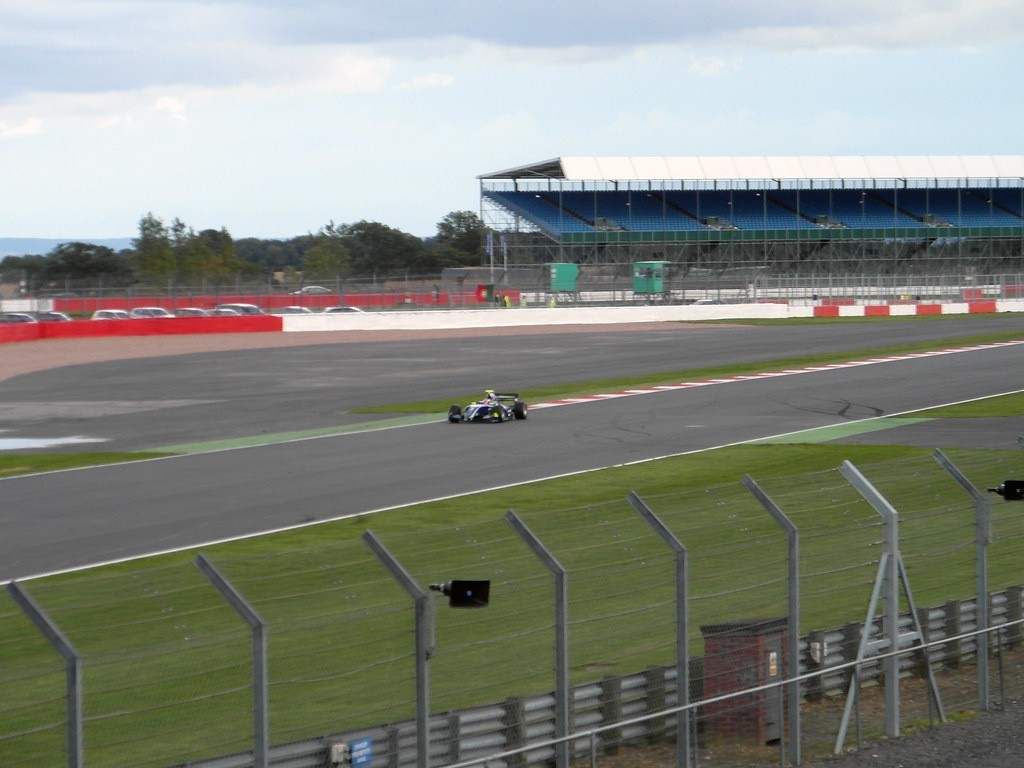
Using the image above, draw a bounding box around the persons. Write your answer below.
[550,296,555,308]
[495,294,511,307]
[487,394,496,401]
[520,296,527,307]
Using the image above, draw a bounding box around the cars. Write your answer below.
[175,308,208,314]
[206,309,240,315]
[323,307,366,312]
[0,312,36,323]
[90,309,135,321]
[289,285,332,295]
[131,306,174,318]
[216,304,267,313]
[271,306,314,315]
[33,310,72,323]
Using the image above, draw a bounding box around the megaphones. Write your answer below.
[429,579,490,608]
[986,480,1024,501]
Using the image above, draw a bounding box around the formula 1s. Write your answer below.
[449,388,528,424]
